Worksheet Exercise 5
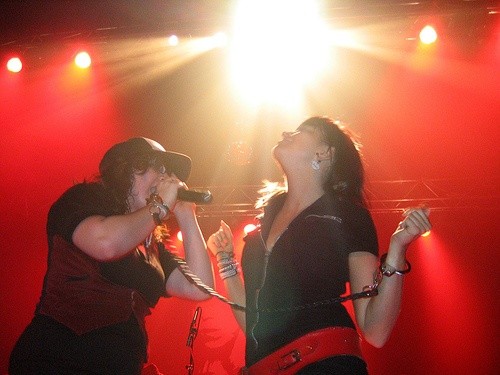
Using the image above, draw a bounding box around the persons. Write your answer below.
[8,137,216,375]
[206,118,432,375]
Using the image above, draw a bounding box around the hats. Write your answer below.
[98,136,192,183]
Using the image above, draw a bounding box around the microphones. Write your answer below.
[177,189,213,204]
[186,307,200,346]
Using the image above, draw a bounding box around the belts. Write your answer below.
[237,326,368,375]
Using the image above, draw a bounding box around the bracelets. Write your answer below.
[215,251,239,280]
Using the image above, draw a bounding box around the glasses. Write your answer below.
[151,158,172,175]
